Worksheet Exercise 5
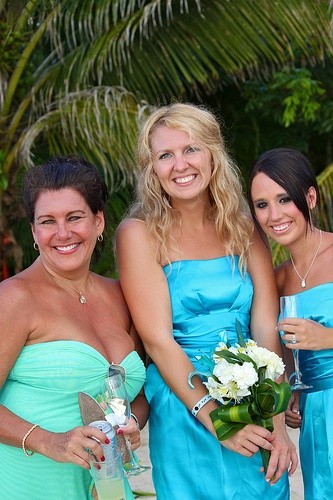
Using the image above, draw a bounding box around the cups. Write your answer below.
[89,446,126,500]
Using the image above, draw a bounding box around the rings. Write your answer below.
[290,333,296,344]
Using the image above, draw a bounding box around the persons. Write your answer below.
[112,101,299,500]
[245,146,332,500]
[0,153,153,500]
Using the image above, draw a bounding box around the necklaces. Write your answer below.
[289,229,324,288]
[38,255,91,304]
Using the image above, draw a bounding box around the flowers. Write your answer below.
[193,317,291,482]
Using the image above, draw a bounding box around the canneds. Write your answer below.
[85,420,118,479]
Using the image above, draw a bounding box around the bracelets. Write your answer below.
[22,422,41,458]
[190,392,216,416]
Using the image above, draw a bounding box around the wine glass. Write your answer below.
[278,293,314,391]
[103,373,152,477]
